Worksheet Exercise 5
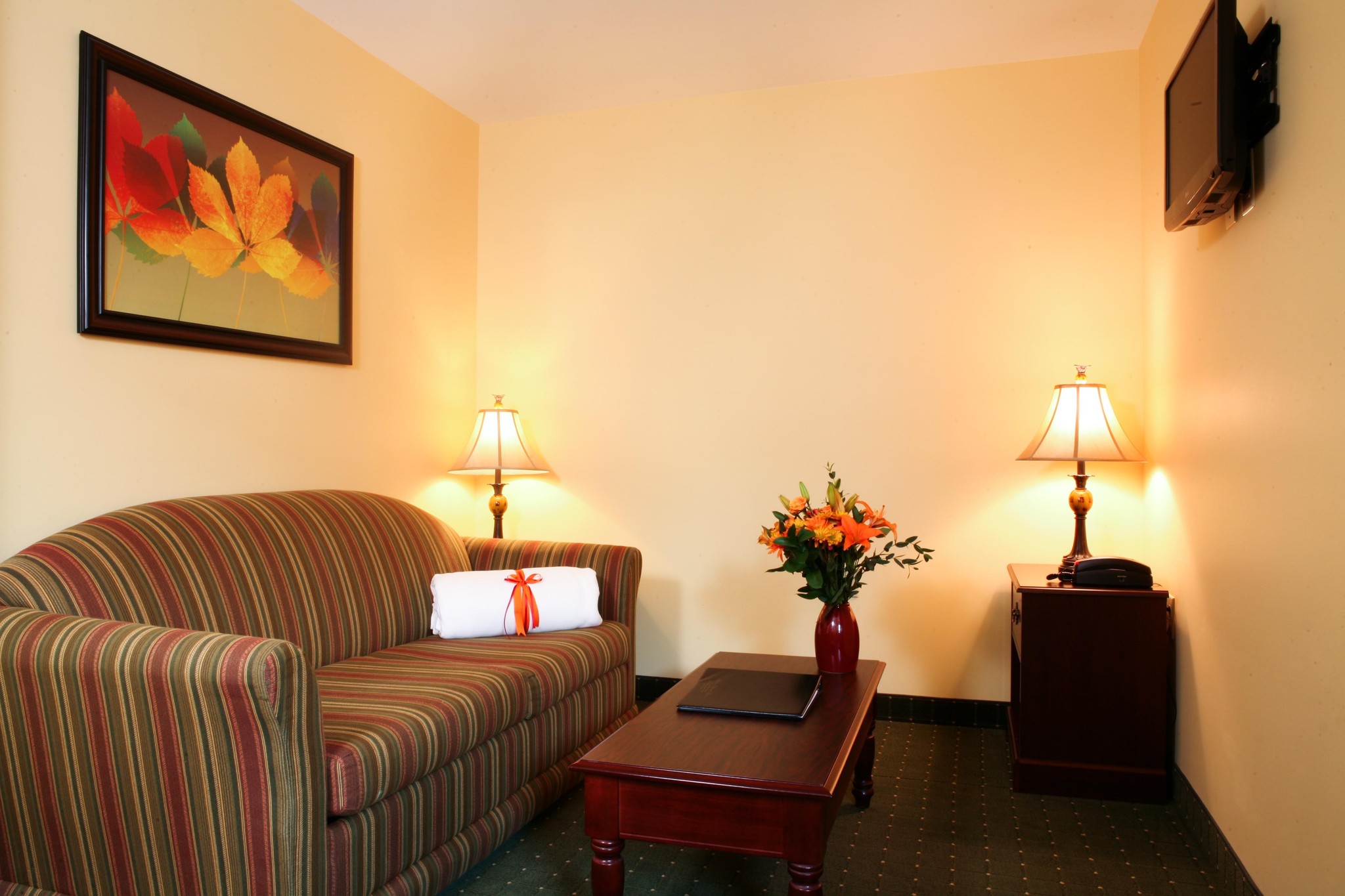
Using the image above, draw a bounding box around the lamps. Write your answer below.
[1016,364,1148,572]
[448,395,550,539]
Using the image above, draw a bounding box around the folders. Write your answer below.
[676,667,821,720]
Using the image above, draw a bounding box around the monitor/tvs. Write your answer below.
[1164,1,1256,231]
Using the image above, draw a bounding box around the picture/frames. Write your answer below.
[78,29,352,365]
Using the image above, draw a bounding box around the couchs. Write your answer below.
[0,490,642,896]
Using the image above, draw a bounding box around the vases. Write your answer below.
[815,602,860,674]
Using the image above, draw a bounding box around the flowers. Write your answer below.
[758,462,935,624]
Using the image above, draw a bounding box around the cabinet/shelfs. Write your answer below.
[1006,562,1168,795]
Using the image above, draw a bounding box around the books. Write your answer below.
[675,666,825,718]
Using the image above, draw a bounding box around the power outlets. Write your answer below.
[1166,594,1175,619]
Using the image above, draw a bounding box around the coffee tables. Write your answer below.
[570,652,886,896]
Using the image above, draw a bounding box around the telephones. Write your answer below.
[1072,557,1153,590]
